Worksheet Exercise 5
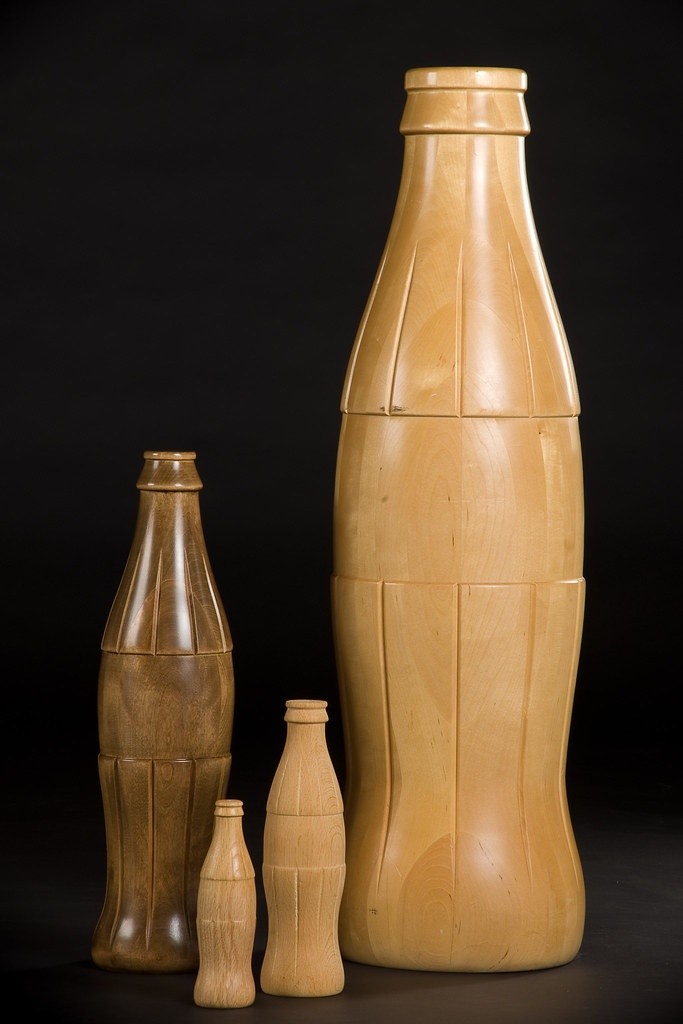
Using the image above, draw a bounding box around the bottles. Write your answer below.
[329,61,587,973]
[194,798,260,1008]
[93,450,239,973]
[257,697,348,998]
